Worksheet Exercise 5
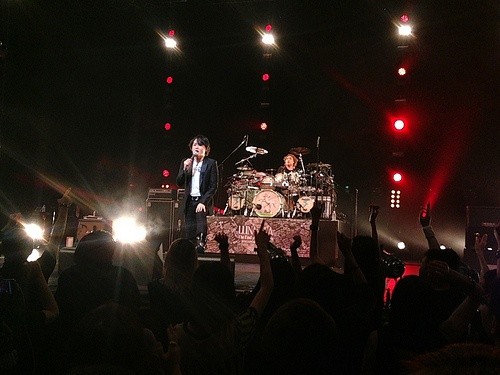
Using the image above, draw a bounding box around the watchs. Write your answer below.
[495,250,500,258]
[59,201,70,209]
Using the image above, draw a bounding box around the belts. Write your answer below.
[188,196,202,202]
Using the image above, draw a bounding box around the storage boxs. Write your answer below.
[145,189,185,255]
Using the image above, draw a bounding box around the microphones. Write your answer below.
[188,150,198,165]
[245,135,248,147]
[317,136,320,147]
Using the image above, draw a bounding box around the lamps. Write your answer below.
[382,249,406,278]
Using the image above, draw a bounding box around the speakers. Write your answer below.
[76,220,112,240]
[464,205,500,265]
[144,200,180,251]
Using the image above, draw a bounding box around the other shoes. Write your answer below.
[195,244,205,254]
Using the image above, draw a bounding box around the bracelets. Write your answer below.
[309,225,319,231]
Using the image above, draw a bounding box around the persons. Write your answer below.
[176,134,220,254]
[277,153,300,174]
[0,188,500,375]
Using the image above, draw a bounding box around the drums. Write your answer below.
[282,172,301,196]
[229,195,245,210]
[236,187,259,209]
[296,197,314,214]
[275,172,290,189]
[261,177,274,189]
[253,189,286,218]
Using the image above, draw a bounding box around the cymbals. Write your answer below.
[289,147,312,155]
[246,146,268,155]
[237,167,252,170]
[245,171,266,177]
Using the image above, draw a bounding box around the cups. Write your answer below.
[66,237,73,247]
